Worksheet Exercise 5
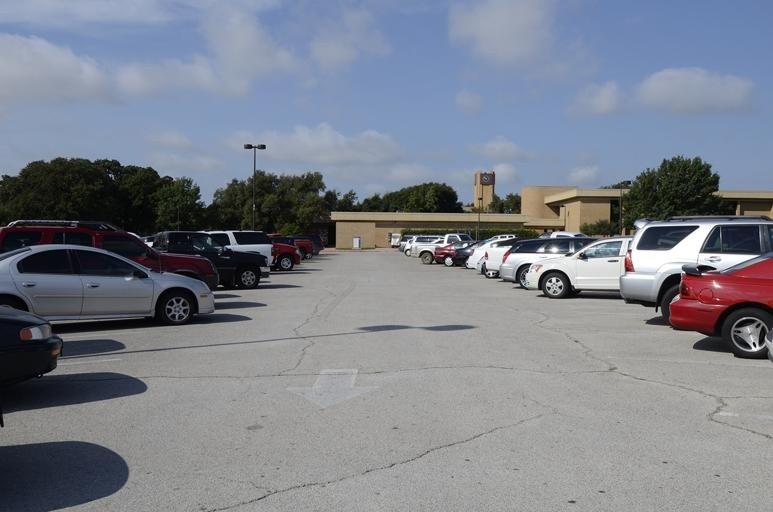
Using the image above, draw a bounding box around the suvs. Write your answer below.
[617,214,773,323]
[0,217,219,294]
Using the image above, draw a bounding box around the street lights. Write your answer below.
[244,143,267,228]
[617,180,631,232]
[477,197,482,240]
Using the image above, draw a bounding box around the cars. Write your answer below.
[524,236,679,298]
[666,248,773,361]
[0,243,217,329]
[388,229,606,290]
[0,302,64,395]
[132,229,324,290]
